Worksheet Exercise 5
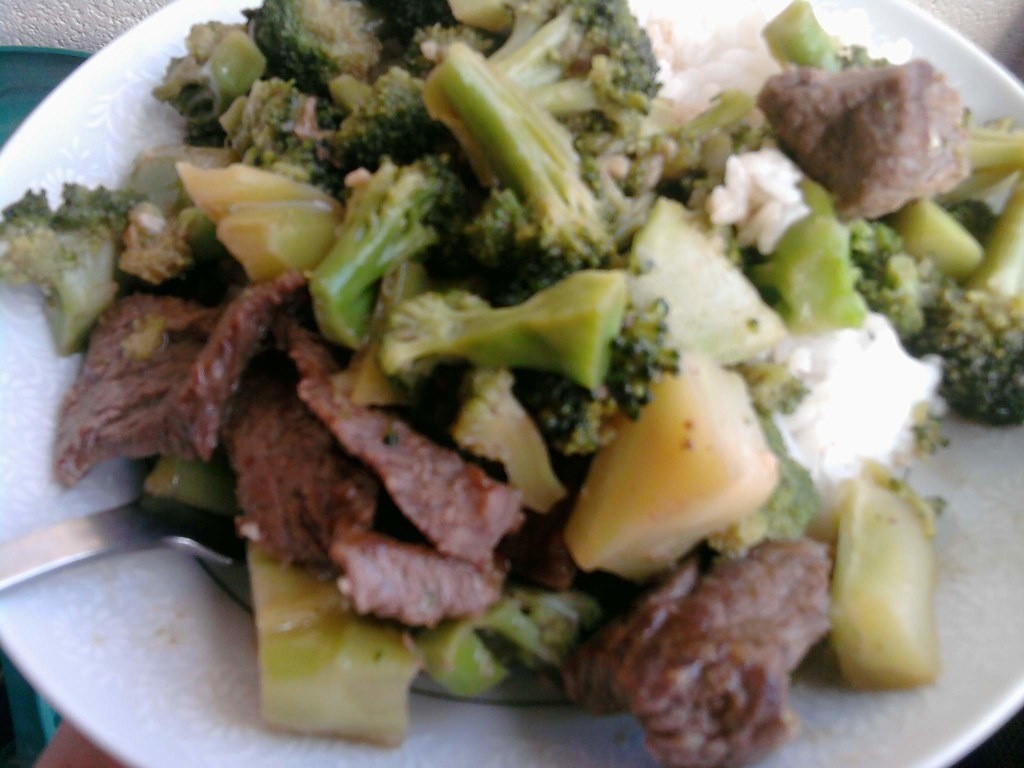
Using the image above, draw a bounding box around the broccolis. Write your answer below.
[1,0,1022,704]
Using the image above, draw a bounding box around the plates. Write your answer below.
[1,0,1024,767]
